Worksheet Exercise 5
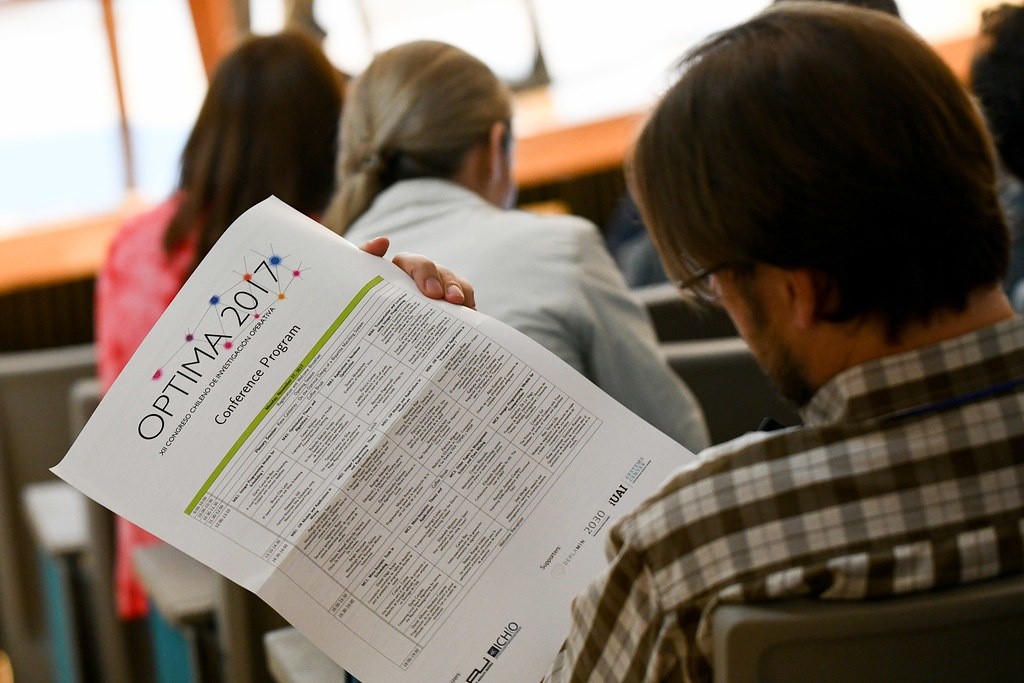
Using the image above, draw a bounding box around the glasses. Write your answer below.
[675,258,730,313]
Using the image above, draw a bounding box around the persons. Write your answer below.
[97,32,350,683]
[321,41,708,458]
[357,0,1024,683]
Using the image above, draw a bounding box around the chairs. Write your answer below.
[0,274,1024,683]
